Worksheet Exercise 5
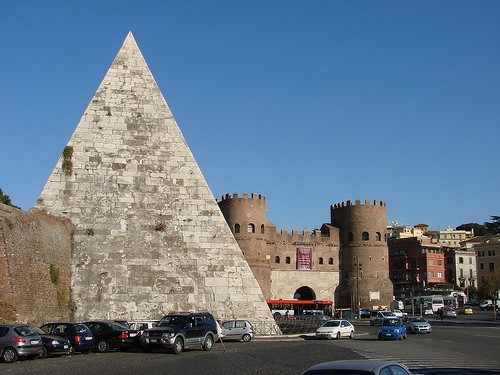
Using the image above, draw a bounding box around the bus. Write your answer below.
[411,296,444,312]
[442,296,458,309]
[266,300,333,320]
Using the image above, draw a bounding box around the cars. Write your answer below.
[12,324,74,357]
[479,304,499,311]
[1,324,42,362]
[458,306,473,314]
[38,323,97,354]
[465,300,481,307]
[335,309,404,320]
[417,306,433,315]
[316,319,355,339]
[81,319,141,353]
[401,315,432,334]
[377,317,407,340]
[213,320,255,342]
[441,308,457,318]
[300,359,412,375]
[369,310,398,326]
[138,311,219,353]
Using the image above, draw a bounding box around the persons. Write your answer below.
[436,307,444,322]
